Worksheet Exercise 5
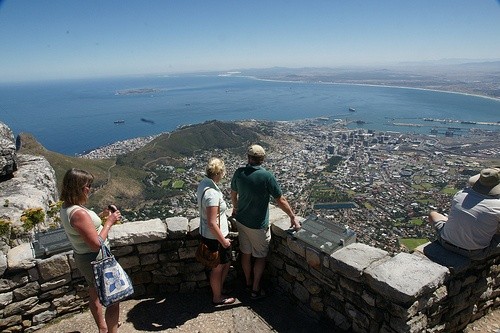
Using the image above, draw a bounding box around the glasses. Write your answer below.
[85,185,91,189]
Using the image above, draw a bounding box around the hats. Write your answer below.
[469,166,500,195]
[247,145,266,157]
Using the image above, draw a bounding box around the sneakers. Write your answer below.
[214,295,235,304]
[251,287,260,297]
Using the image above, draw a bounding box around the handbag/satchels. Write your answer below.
[194,243,222,268]
[91,235,134,307]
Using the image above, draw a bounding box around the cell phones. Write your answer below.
[109,205,117,213]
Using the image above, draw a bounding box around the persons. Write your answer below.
[60,167,122,333]
[230,145,301,300]
[197,157,235,305]
[429,168,500,262]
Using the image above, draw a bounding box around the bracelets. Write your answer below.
[104,223,111,228]
